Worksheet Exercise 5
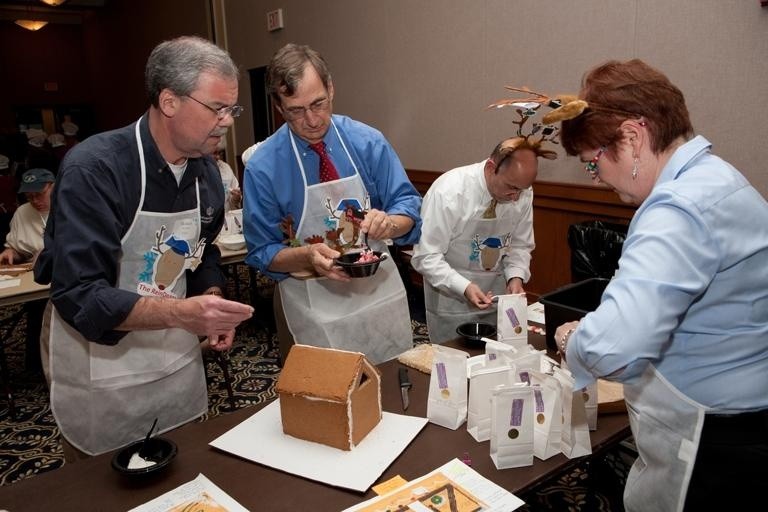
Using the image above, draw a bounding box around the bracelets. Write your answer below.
[207,290,225,299]
[561,328,575,354]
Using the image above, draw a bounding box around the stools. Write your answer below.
[198,334,236,412]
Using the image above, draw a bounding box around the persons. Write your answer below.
[242,43,423,372]
[211,135,242,214]
[33,36,255,466]
[551,59,767,512]
[0,167,55,380]
[411,136,538,345]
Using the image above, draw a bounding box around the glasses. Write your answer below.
[285,92,331,121]
[213,102,251,121]
[26,184,51,201]
[582,120,646,175]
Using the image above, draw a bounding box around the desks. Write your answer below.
[0,227,264,420]
[1,310,639,512]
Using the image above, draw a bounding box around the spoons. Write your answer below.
[362,232,371,255]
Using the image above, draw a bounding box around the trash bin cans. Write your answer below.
[568,221,628,283]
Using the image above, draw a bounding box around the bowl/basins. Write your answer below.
[219,234,247,251]
[111,435,181,476]
[456,322,497,345]
[335,249,389,279]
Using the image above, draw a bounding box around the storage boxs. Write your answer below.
[537,277,612,359]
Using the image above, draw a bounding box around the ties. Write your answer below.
[307,141,341,186]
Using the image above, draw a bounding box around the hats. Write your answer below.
[17,166,59,197]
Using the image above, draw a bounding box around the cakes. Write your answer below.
[274,344,383,452]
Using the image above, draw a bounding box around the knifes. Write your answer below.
[398,367,412,409]
